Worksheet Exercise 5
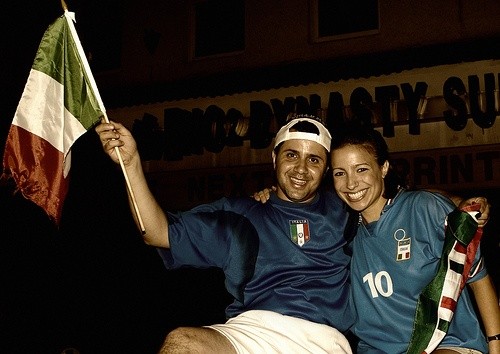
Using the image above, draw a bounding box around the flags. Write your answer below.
[0,10,107,231]
[405,201,486,353]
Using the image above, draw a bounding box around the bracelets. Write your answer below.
[486,334,500,343]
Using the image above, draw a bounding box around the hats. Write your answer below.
[274,118,331,153]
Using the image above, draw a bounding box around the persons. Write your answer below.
[95,113,491,354]
[248,127,500,354]
